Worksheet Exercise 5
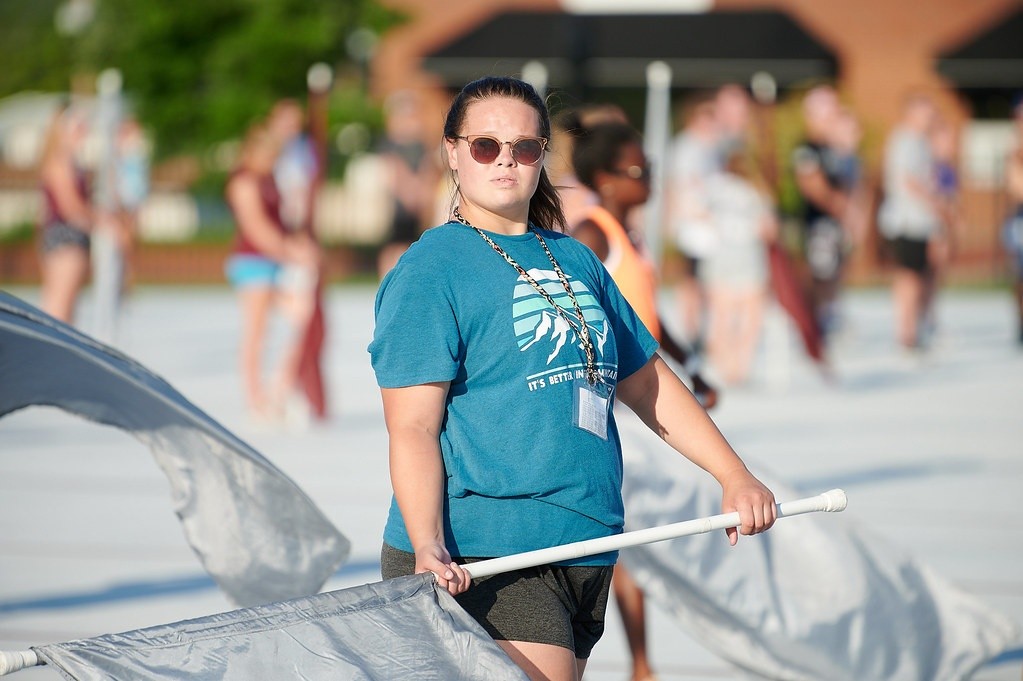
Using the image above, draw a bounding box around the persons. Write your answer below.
[42,102,151,334]
[562,114,718,679]
[366,77,775,680]
[665,81,1023,382]
[228,104,325,422]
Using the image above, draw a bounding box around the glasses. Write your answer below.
[607,166,650,185]
[448,135,547,165]
